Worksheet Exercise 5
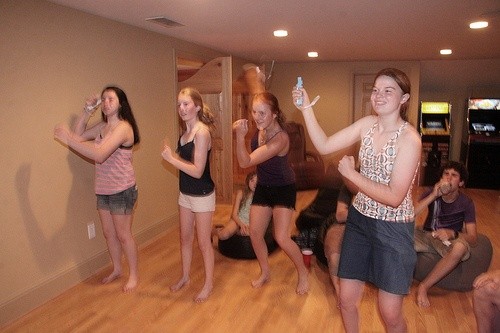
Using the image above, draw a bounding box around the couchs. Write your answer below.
[281,122,325,190]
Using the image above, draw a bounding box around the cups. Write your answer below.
[302,250,313,267]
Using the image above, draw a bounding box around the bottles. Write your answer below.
[440,178,450,195]
[425,140,442,184]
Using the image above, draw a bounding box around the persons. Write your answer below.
[292,67,421,333]
[55,86,139,294]
[471,269,500,333]
[234,64,312,295]
[293,160,362,313]
[411,161,478,310]
[211,172,257,241]
[161,88,224,304]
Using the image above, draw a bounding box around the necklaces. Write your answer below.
[259,125,281,143]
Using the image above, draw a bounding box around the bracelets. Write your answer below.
[452,228,459,240]
[84,105,93,117]
